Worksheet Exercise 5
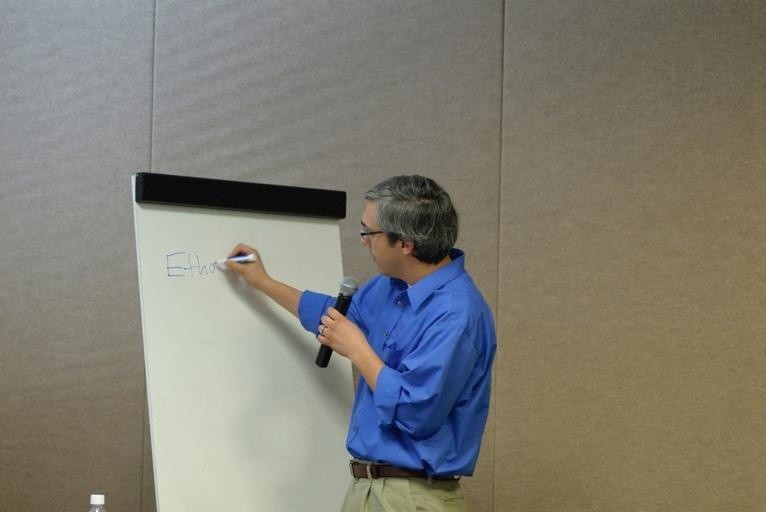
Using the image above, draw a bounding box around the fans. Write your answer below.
[131,173,356,511]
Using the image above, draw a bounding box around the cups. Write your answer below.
[360,227,383,242]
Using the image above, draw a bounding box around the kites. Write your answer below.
[215,254,256,265]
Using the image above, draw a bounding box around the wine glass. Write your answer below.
[319,327,325,336]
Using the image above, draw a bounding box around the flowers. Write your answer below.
[316,278,359,368]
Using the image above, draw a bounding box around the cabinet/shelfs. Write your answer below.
[88,495,106,512]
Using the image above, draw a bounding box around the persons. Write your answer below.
[224,174,497,512]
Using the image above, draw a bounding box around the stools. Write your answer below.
[349,459,455,481]
